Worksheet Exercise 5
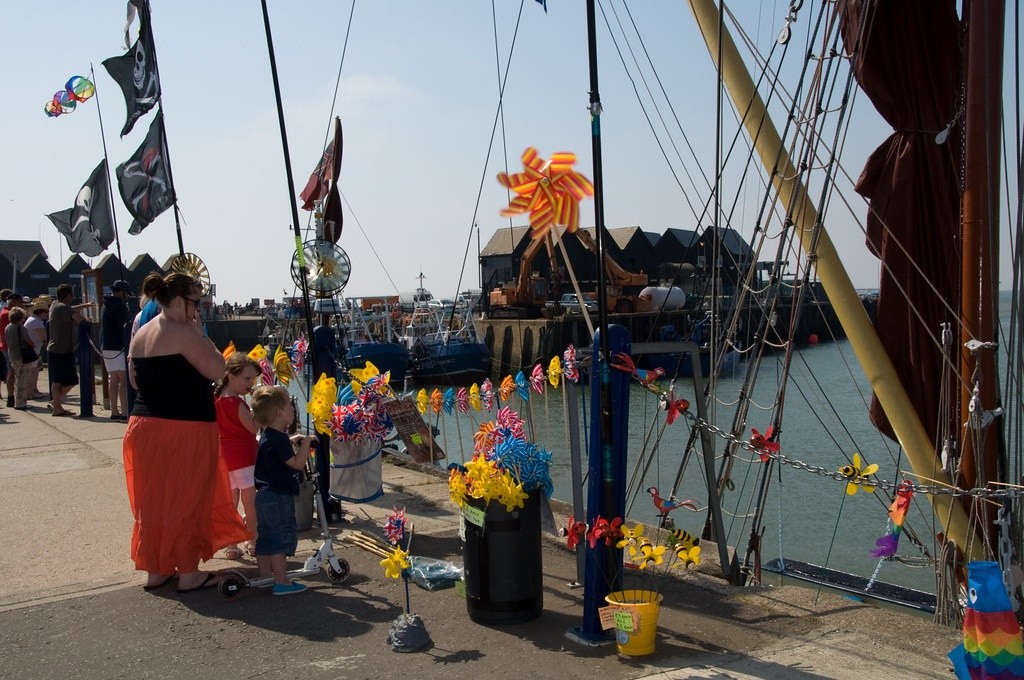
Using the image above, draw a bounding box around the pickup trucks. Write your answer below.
[545,293,598,314]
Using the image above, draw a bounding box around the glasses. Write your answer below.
[181,295,201,308]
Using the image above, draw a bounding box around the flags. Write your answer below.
[46,158,117,258]
[115,107,176,236]
[300,116,343,248]
[100,0,161,140]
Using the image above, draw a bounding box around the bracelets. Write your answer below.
[201,335,208,338]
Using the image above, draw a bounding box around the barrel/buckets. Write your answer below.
[329,434,382,503]
[295,481,315,531]
[462,489,544,623]
[605,589,664,656]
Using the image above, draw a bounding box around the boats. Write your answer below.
[258,271,489,387]
[653,310,742,378]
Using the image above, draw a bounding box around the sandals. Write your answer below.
[248,544,256,556]
[226,547,244,559]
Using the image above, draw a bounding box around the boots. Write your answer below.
[6,395,15,407]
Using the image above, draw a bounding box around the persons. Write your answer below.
[250,386,319,595]
[122,273,255,594]
[213,353,263,560]
[0,273,165,423]
[196,298,290,321]
[345,300,400,316]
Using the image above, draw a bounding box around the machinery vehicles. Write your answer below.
[488,220,651,320]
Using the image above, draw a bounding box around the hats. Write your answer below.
[31,294,52,305]
[22,296,33,305]
[109,280,134,296]
[6,294,23,300]
[29,301,48,314]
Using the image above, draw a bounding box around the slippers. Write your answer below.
[175,573,218,594]
[142,570,179,590]
[52,410,76,416]
[47,405,53,413]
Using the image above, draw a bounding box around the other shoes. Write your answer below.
[120,416,128,423]
[110,414,121,421]
[16,405,32,409]
[271,581,308,596]
[32,388,48,398]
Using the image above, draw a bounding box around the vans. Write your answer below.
[399,288,434,312]
[428,298,455,312]
